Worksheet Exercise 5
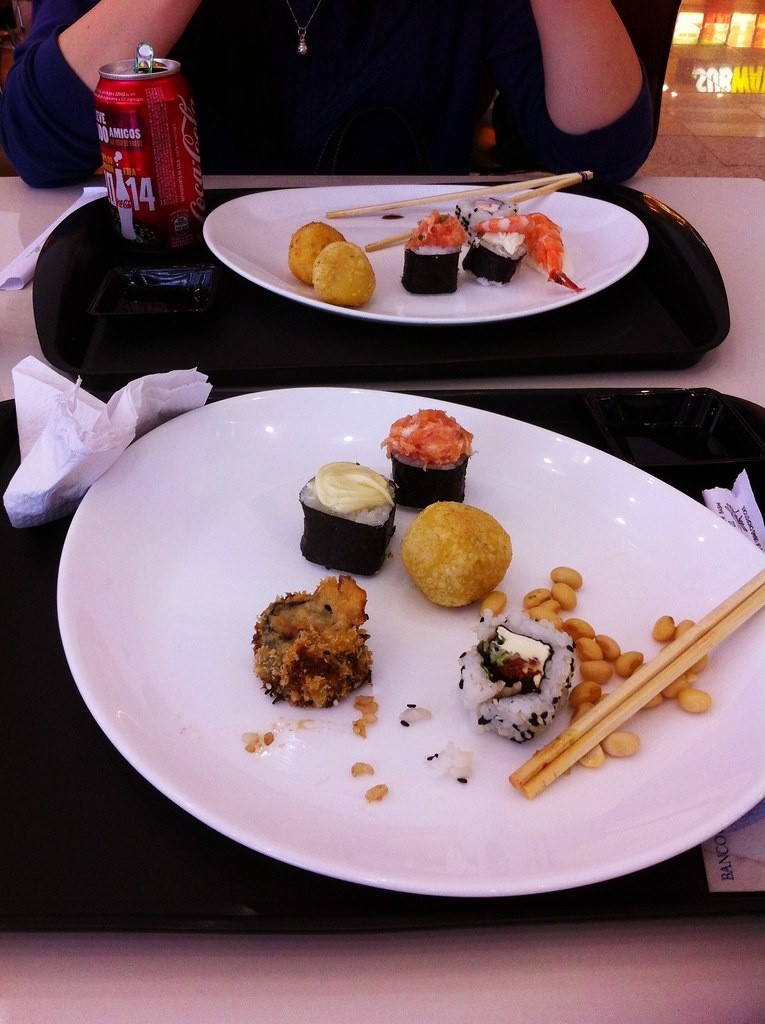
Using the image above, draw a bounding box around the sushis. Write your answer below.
[399,192,583,299]
[455,608,576,743]
[295,408,479,577]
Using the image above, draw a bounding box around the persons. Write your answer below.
[0,0,686,194]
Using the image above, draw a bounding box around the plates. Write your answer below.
[54,385,765,896]
[198,182,651,326]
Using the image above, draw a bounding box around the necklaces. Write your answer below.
[284,0,326,56]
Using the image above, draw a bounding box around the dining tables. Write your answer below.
[1,174,765,1024]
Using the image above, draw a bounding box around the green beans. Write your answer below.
[481,566,714,771]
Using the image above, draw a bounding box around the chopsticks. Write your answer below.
[327,167,597,250]
[512,559,765,799]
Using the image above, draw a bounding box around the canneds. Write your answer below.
[94,39,206,254]
